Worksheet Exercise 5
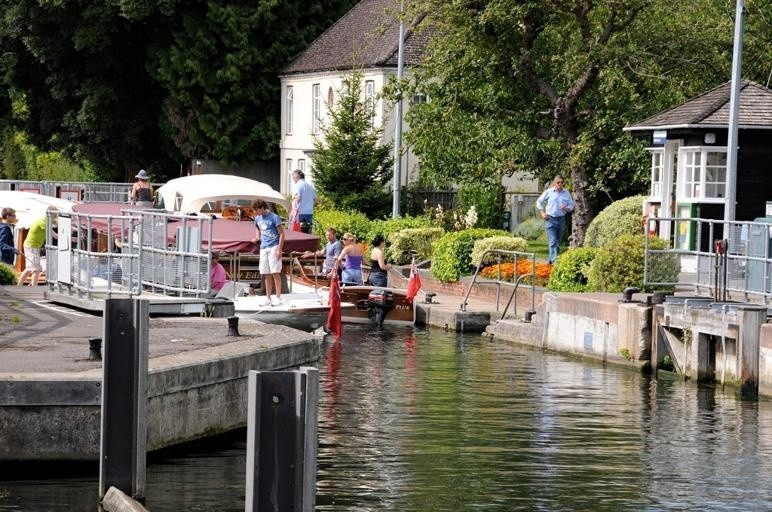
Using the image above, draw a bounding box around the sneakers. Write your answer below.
[259,299,272,307]
[271,298,283,307]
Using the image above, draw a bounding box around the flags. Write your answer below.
[405,259,422,304]
[326,338,344,424]
[327,267,342,339]
[404,335,417,414]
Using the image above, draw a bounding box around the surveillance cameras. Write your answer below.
[196,161,201,168]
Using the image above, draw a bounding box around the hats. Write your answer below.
[134,169,151,180]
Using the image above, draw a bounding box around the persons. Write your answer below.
[250,198,285,307]
[18,205,83,286]
[369,331,388,419]
[327,232,363,286]
[288,170,318,234]
[131,169,153,206]
[301,227,343,279]
[536,174,574,264]
[369,235,392,287]
[1,207,22,269]
[200,251,226,299]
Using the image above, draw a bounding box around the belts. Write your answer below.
[548,215,565,221]
